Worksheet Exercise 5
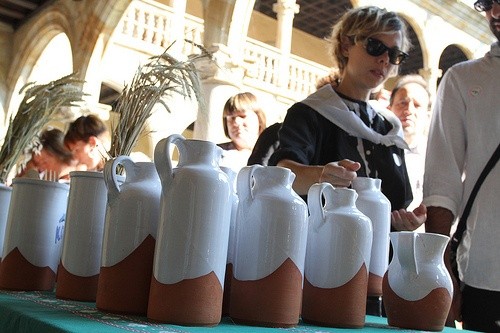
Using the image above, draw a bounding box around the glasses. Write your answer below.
[361,35,409,66]
[473,0,500,12]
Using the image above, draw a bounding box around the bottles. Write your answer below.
[146,134,231,327]
[349,177,391,296]
[219,165,239,317]
[302,180,374,329]
[56,170,126,301]
[381,231,454,331]
[96,156,162,315]
[1,178,69,292]
[0,183,13,262]
[230,164,309,328]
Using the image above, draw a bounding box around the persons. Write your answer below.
[247,70,383,166]
[62,114,152,177]
[267,6,427,317]
[40,128,86,183]
[216,92,269,195]
[386,74,465,328]
[422,0,500,333]
[21,143,45,180]
